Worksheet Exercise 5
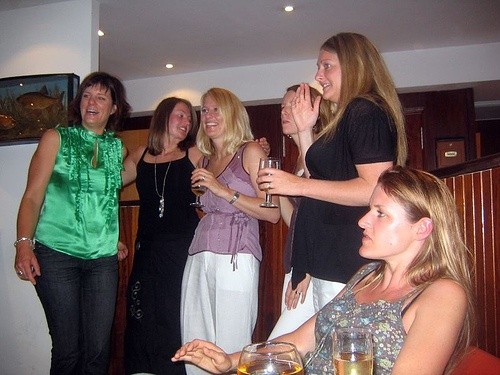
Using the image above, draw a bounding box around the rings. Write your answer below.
[202,176,205,181]
[17,271,22,275]
[269,183,270,188]
[295,291,300,294]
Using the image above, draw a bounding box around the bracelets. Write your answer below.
[14,237,32,247]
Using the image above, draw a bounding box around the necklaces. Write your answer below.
[154,156,171,218]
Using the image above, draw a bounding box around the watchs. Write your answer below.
[229,191,240,204]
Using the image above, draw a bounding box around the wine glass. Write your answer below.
[259,157,280,207]
[190,179,206,207]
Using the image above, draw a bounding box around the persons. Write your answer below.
[13,71,133,375]
[121,97,271,375]
[178,88,281,375]
[257,31,412,342]
[170,164,479,375]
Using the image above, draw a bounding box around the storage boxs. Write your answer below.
[0,73,79,146]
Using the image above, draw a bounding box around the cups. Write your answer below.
[237,342,304,375]
[333,326,374,375]
